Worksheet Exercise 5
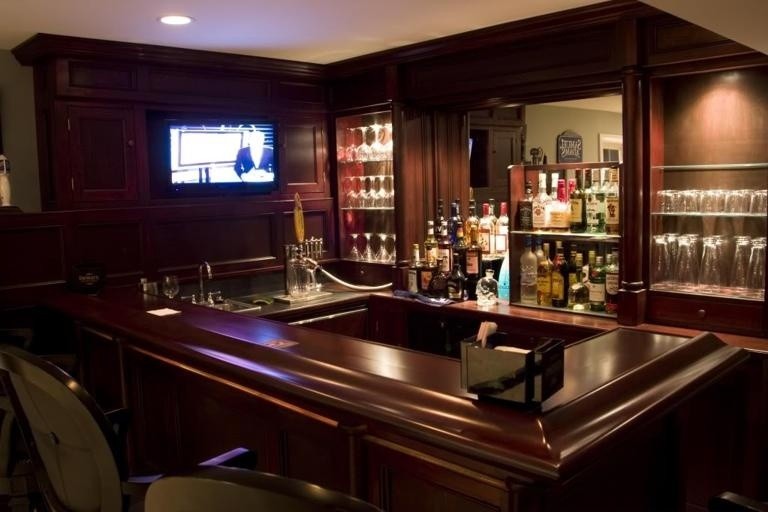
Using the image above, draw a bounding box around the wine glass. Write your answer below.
[344,174,394,208]
[347,232,397,264]
[344,124,393,162]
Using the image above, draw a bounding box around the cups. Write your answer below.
[651,232,767,300]
[285,262,310,298]
[161,276,179,300]
[143,282,158,297]
[653,189,768,216]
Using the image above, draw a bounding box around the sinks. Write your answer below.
[209,298,262,314]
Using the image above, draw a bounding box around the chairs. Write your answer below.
[0,337,252,512]
[135,455,391,511]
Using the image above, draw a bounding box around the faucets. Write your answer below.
[197,260,213,297]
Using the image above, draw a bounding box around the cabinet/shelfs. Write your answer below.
[465,104,529,229]
[502,159,628,323]
[34,94,143,209]
[618,51,767,339]
[272,108,335,202]
[335,97,473,286]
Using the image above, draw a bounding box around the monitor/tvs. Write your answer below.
[163,116,278,193]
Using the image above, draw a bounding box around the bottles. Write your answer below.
[407,197,509,303]
[476,268,498,306]
[518,165,619,312]
